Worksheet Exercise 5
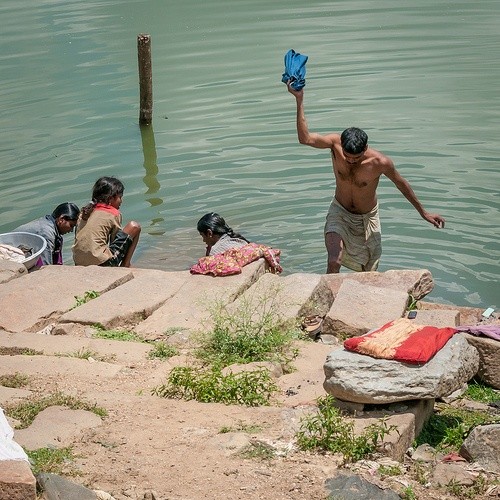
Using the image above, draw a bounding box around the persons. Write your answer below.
[286,79,446,273]
[197,212,251,256]
[71,176,141,267]
[10,203,80,267]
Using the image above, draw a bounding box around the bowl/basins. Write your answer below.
[0,232,47,269]
